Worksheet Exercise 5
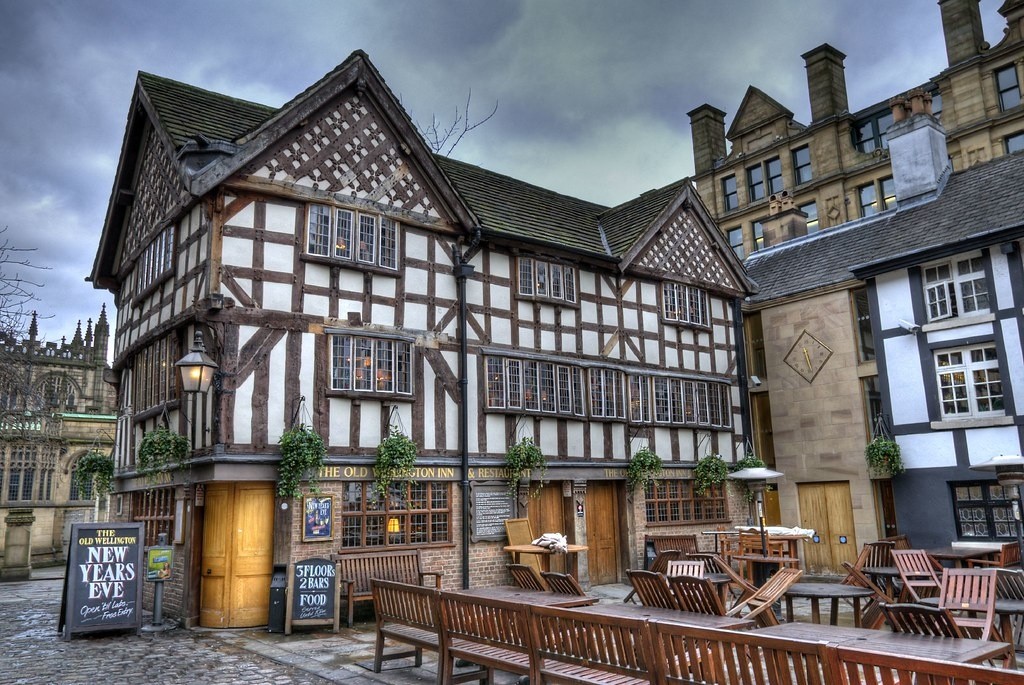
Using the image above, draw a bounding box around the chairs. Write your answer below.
[624,549,682,605]
[712,553,781,618]
[666,575,751,679]
[890,548,942,603]
[967,541,1020,568]
[931,567,1009,669]
[974,566,1024,644]
[540,570,593,607]
[879,601,964,639]
[878,534,940,565]
[725,567,804,629]
[862,541,900,597]
[841,545,880,616]
[841,561,913,629]
[665,562,704,610]
[505,563,549,592]
[737,527,769,579]
[686,552,737,599]
[626,569,679,610]
[717,526,746,562]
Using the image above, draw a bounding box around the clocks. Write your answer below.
[783,329,834,384]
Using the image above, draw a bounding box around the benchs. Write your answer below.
[434,589,585,685]
[647,617,882,685]
[329,549,442,629]
[644,534,700,554]
[826,642,1024,685]
[524,603,699,685]
[369,577,486,685]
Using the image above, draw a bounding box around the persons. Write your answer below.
[159,562,171,579]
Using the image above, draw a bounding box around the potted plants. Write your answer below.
[864,435,905,480]
[137,423,191,493]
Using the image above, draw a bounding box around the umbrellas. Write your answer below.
[529,532,568,553]
[736,523,817,537]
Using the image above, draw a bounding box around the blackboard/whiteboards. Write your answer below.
[63,522,145,632]
[286,557,341,625]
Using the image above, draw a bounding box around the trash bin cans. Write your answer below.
[644,539,658,570]
[268,562,288,634]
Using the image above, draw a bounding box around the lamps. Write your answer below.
[173,331,235,398]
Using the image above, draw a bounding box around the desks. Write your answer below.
[860,566,899,612]
[725,536,810,583]
[568,601,757,656]
[919,596,1024,671]
[503,544,589,589]
[783,582,876,626]
[927,547,1000,569]
[746,621,1010,685]
[733,556,800,616]
[455,585,599,608]
[662,573,731,609]
